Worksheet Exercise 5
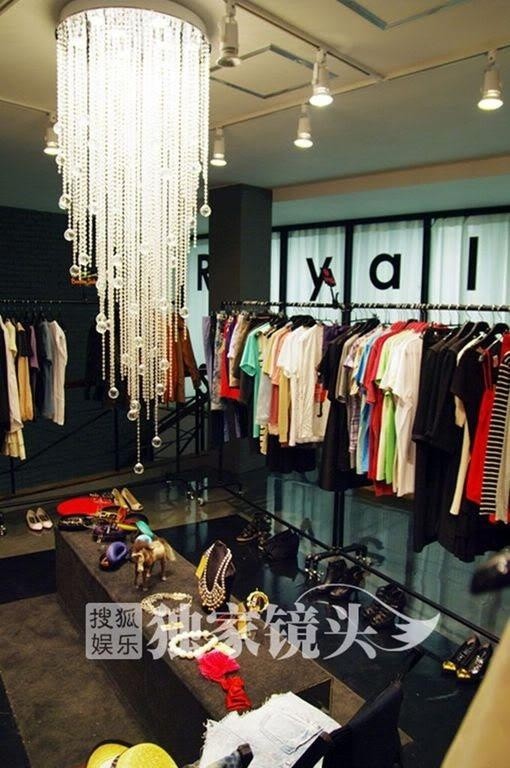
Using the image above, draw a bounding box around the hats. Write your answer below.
[85,739,179,768]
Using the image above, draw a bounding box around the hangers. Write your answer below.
[0,298,63,327]
[217,299,509,362]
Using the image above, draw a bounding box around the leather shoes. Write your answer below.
[26,510,43,531]
[317,559,363,598]
[36,508,53,529]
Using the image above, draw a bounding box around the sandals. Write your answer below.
[121,487,144,511]
[359,583,407,627]
[112,488,131,513]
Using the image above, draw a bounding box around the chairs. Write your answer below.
[201,646,426,768]
[209,743,253,767]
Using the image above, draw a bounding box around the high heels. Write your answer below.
[457,642,492,679]
[442,633,481,670]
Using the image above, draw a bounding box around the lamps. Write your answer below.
[52,1,211,476]
[214,1,333,107]
[44,110,57,155]
[209,47,503,167]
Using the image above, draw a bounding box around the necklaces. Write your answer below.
[195,542,234,613]
[140,590,194,618]
[168,628,219,660]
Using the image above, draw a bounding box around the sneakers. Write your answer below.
[258,529,299,560]
[236,512,272,550]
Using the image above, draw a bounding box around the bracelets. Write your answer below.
[245,590,270,615]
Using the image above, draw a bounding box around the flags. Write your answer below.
[129,537,177,592]
